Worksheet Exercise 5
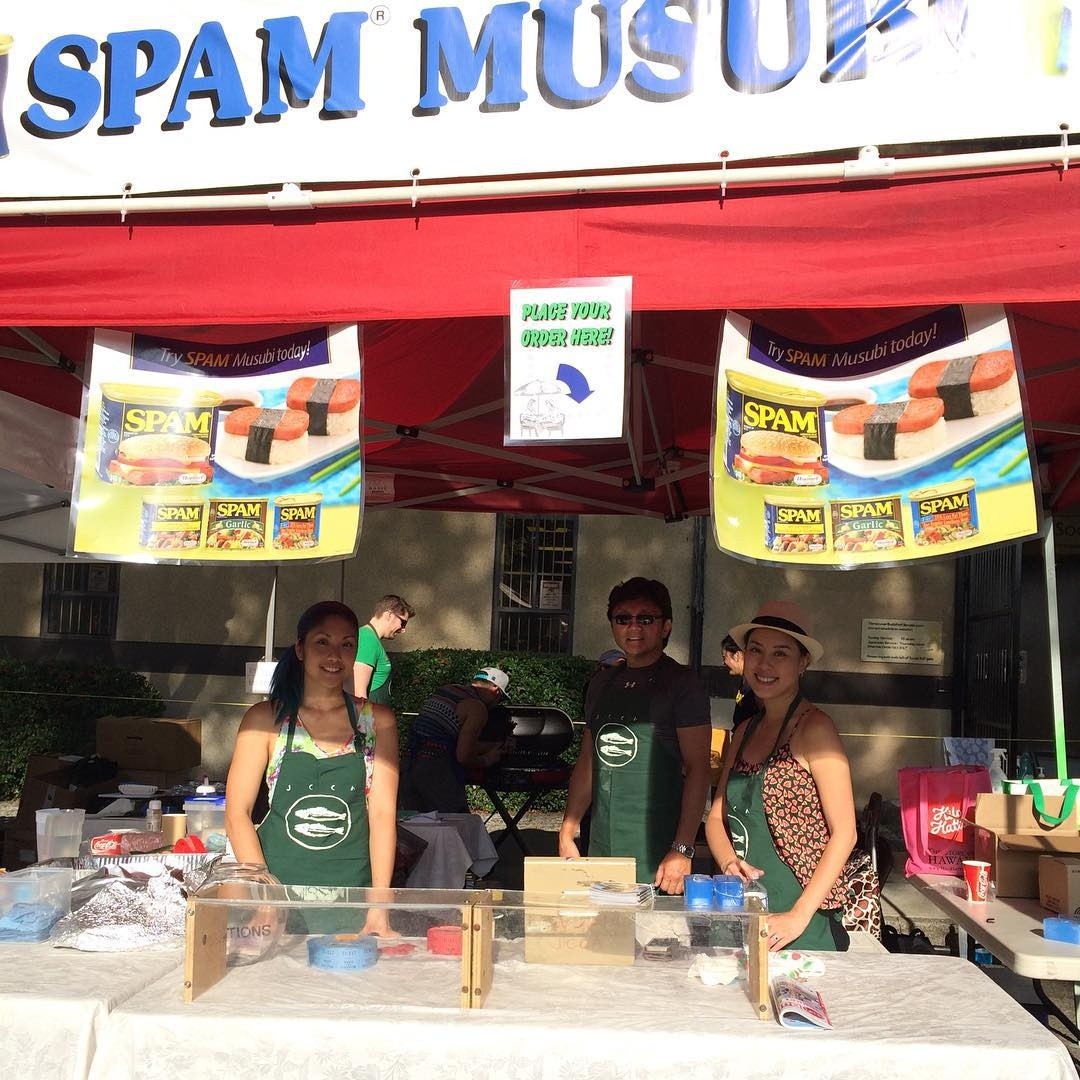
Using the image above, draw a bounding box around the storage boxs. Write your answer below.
[945,794,1080,897]
[98,766,202,791]
[1038,854,1079,917]
[96,716,202,772]
[183,797,226,847]
[1,752,119,871]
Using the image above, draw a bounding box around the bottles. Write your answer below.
[742,872,769,949]
[146,799,163,832]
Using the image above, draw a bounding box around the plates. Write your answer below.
[211,394,359,485]
[119,783,158,797]
[820,388,1021,483]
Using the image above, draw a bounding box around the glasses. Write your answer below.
[611,614,666,625]
[393,612,407,628]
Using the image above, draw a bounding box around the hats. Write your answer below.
[726,600,824,664]
[472,667,512,700]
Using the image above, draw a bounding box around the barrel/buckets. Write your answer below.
[188,862,287,968]
[183,794,229,855]
[35,806,87,864]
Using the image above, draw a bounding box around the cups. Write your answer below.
[961,859,991,904]
[91,831,127,856]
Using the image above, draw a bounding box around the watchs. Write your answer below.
[670,841,697,860]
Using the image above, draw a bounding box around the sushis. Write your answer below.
[224,406,310,465]
[285,376,361,436]
[831,396,947,461]
[906,350,1020,421]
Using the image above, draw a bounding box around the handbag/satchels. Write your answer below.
[1002,778,1079,825]
[898,764,994,879]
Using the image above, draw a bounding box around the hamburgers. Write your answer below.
[105,433,213,486]
[732,430,828,487]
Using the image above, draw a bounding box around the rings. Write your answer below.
[774,935,780,943]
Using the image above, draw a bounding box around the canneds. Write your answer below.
[909,477,980,546]
[138,495,206,552]
[271,493,324,553]
[95,382,224,488]
[828,494,906,554]
[205,497,270,553]
[761,494,826,554]
[723,369,830,489]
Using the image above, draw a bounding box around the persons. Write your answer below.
[222,601,408,945]
[705,598,857,955]
[394,665,513,884]
[557,576,713,903]
[347,595,415,722]
[720,632,762,744]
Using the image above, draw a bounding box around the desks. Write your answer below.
[110,934,1080,1080]
[0,942,186,1080]
[402,812,499,889]
[904,873,1080,1040]
